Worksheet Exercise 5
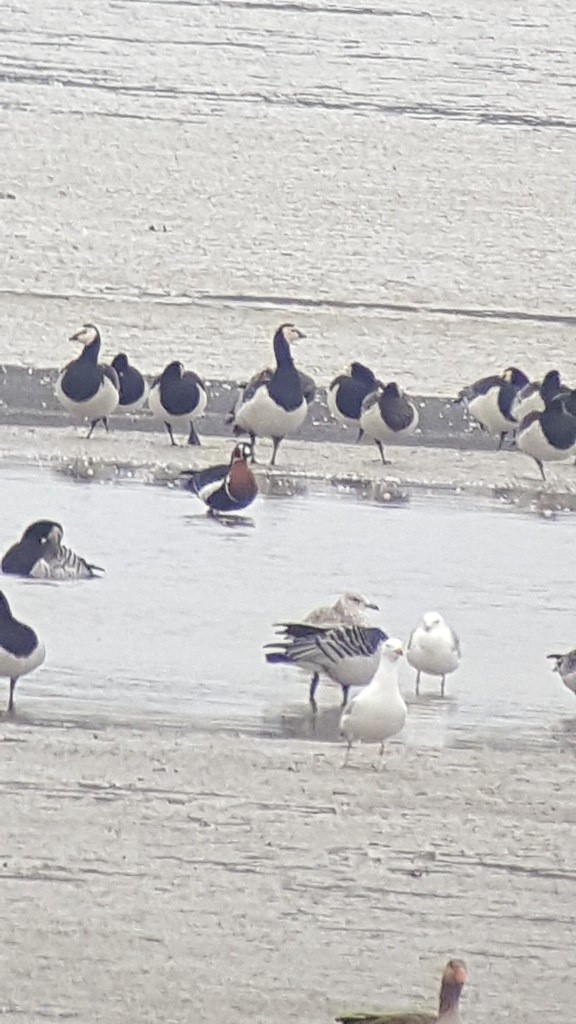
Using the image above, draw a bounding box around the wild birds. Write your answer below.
[113,352,148,414]
[226,323,318,468]
[54,325,120,437]
[335,959,468,1024]
[0,591,49,711]
[511,391,576,479]
[2,520,106,580]
[453,368,531,449]
[302,591,380,706]
[326,361,418,466]
[509,370,573,422]
[339,637,408,750]
[178,441,258,516]
[547,649,576,694]
[148,359,210,446]
[261,623,389,705]
[406,611,460,697]
